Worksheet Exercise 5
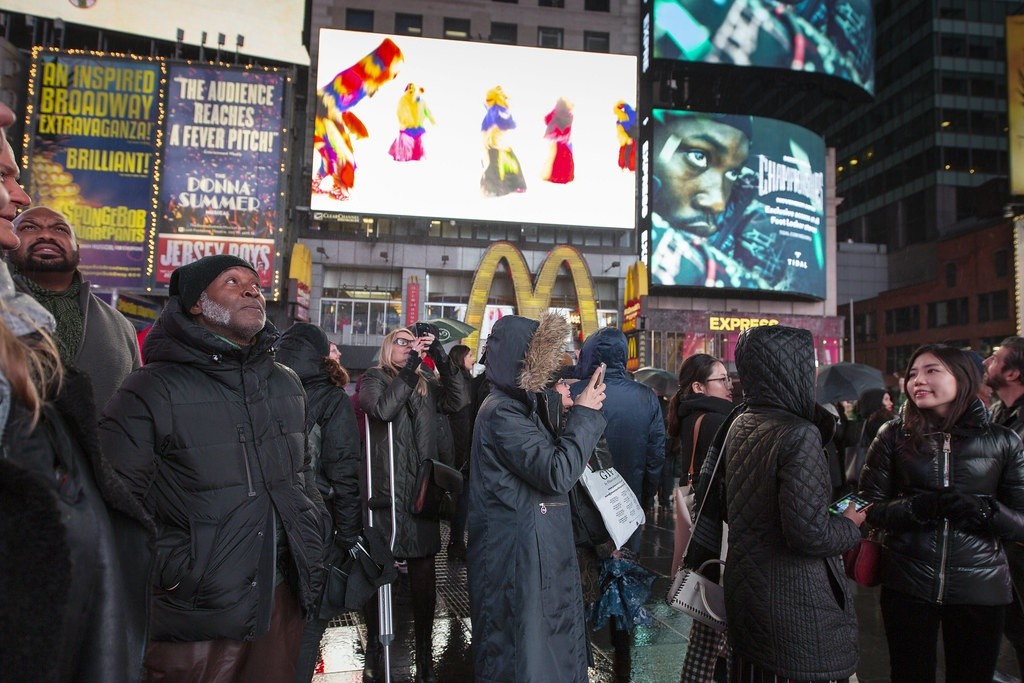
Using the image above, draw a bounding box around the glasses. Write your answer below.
[706,376,733,388]
[393,338,418,348]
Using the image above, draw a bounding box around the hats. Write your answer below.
[859,389,890,412]
[181,255,262,316]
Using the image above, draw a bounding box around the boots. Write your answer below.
[362,638,383,680]
[414,640,436,683]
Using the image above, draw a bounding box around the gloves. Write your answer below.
[944,486,980,518]
[912,485,953,520]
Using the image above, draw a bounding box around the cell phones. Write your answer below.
[827,490,873,520]
[416,322,430,347]
[597,362,607,389]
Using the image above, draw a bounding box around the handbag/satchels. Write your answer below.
[830,444,871,488]
[848,527,885,588]
[669,559,728,631]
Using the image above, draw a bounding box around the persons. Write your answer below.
[97,253,336,683]
[710,165,772,269]
[272,321,364,683]
[0,100,157,682]
[5,207,142,439]
[0,302,102,683]
[651,109,754,242]
[322,314,1024,682]
